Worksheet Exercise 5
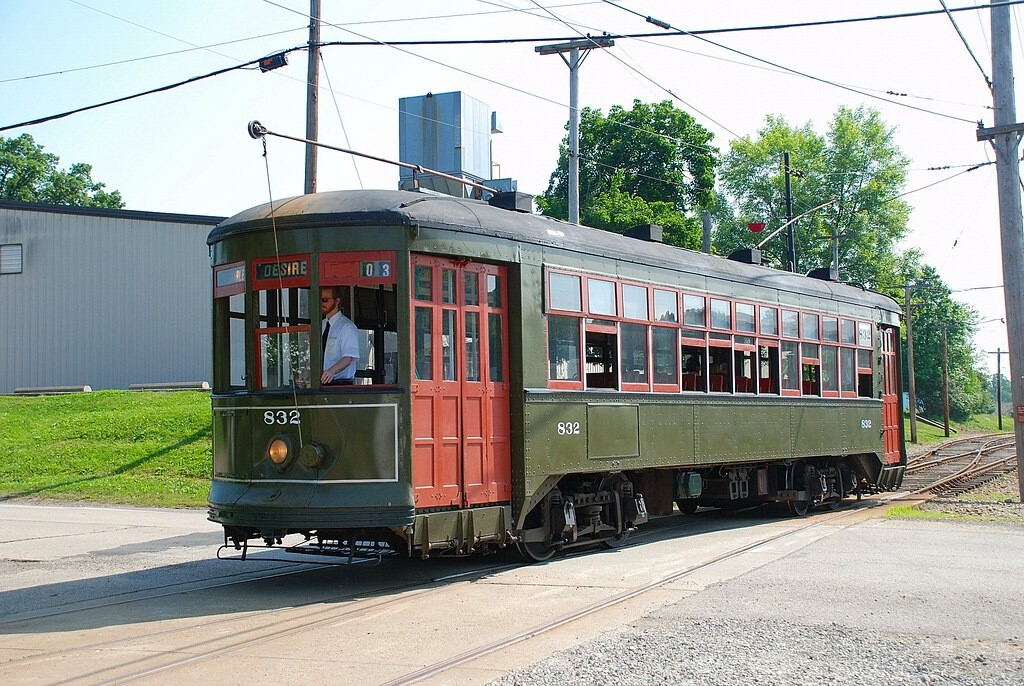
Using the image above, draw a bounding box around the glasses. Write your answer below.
[322,296,339,302]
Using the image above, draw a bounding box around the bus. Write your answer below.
[206,118,907,564]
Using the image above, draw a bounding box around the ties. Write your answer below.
[321,321,330,372]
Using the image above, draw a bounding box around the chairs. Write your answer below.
[585,372,828,395]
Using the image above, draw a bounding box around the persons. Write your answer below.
[685,357,700,374]
[297,286,361,389]
